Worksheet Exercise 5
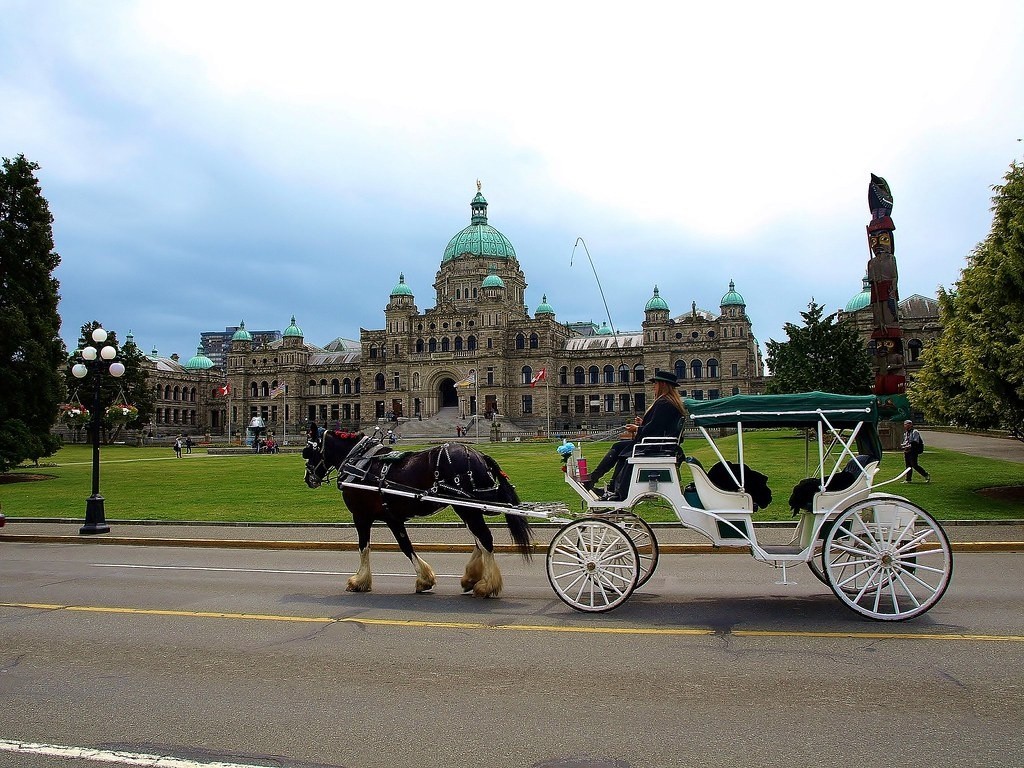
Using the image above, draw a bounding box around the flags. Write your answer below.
[453,373,476,388]
[270,382,284,400]
[219,383,230,396]
[531,368,547,389]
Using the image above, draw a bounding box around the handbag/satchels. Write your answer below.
[910,429,924,454]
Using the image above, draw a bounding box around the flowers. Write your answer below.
[103,404,139,423]
[59,402,92,427]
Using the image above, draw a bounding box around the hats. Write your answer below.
[649,371,682,388]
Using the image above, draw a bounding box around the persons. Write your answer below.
[577,371,687,502]
[185,436,192,454]
[175,436,184,458]
[456,426,461,438]
[899,420,930,484]
[392,434,397,444]
[255,436,279,455]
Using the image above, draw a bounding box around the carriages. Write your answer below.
[302,393,954,622]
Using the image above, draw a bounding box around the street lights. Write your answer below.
[70,327,125,535]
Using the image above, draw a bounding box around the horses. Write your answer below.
[303,422,537,599]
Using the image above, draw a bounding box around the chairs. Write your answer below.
[625,418,686,463]
[812,455,881,515]
[685,456,753,514]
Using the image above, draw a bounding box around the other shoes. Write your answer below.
[582,476,594,491]
[599,492,608,501]
[591,483,614,496]
[606,495,620,501]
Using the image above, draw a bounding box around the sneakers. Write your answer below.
[900,480,911,483]
[926,473,930,484]
